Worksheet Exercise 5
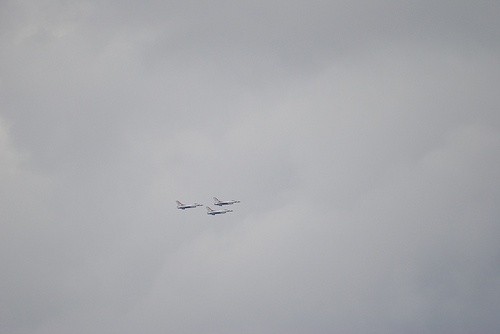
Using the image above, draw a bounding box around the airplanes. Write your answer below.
[213,195,240,207]
[205,206,234,216]
[176,199,204,210]
